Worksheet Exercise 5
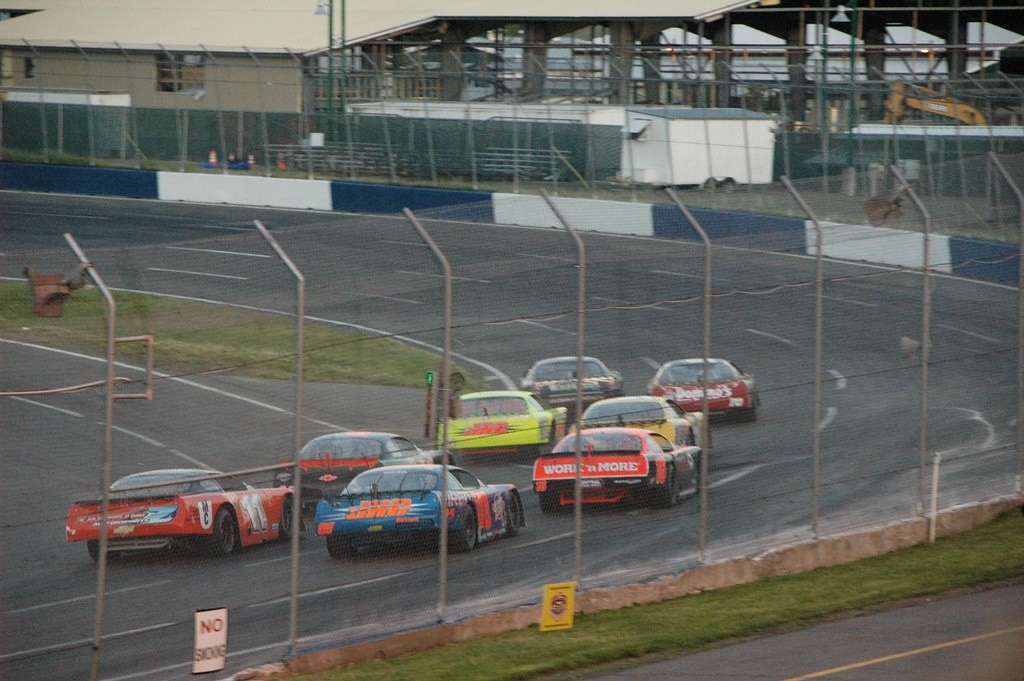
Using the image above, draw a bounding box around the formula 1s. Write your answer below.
[518,355,624,421]
[314,465,526,559]
[646,356,759,423]
[532,428,701,514]
[273,432,450,517]
[438,389,566,463]
[568,396,712,452]
[66,467,301,565]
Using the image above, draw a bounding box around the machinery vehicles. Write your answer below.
[882,80,988,127]
